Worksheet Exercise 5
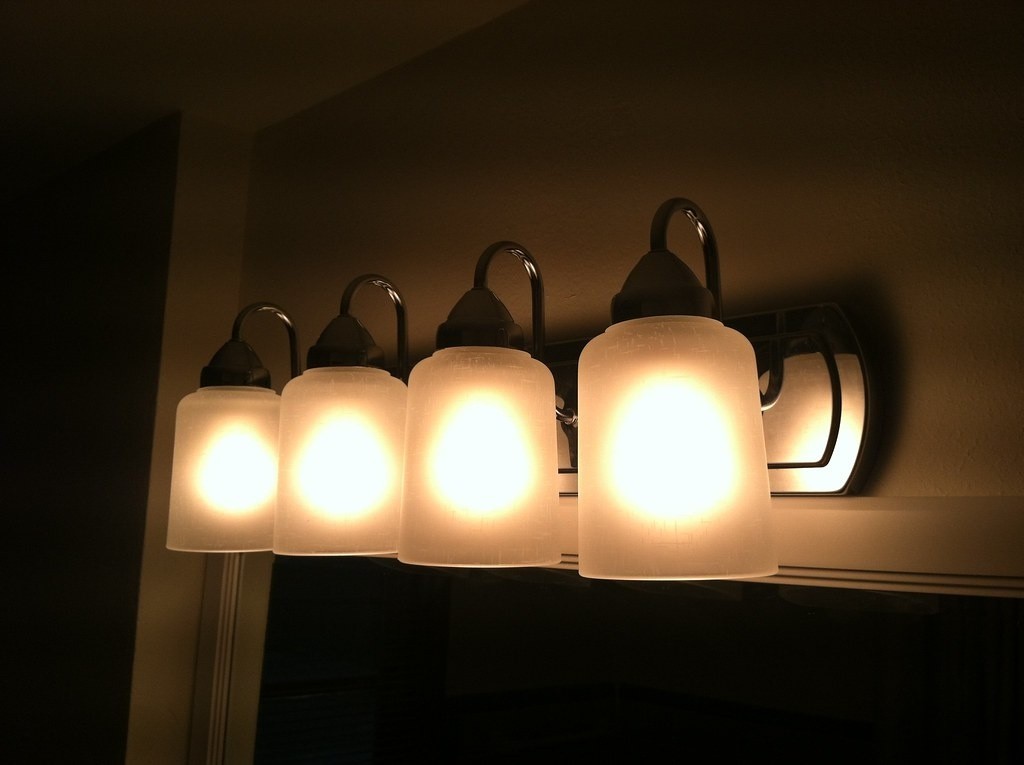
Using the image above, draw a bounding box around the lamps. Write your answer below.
[270,274,407,554]
[576,196,873,581]
[398,241,577,567]
[167,304,299,552]
